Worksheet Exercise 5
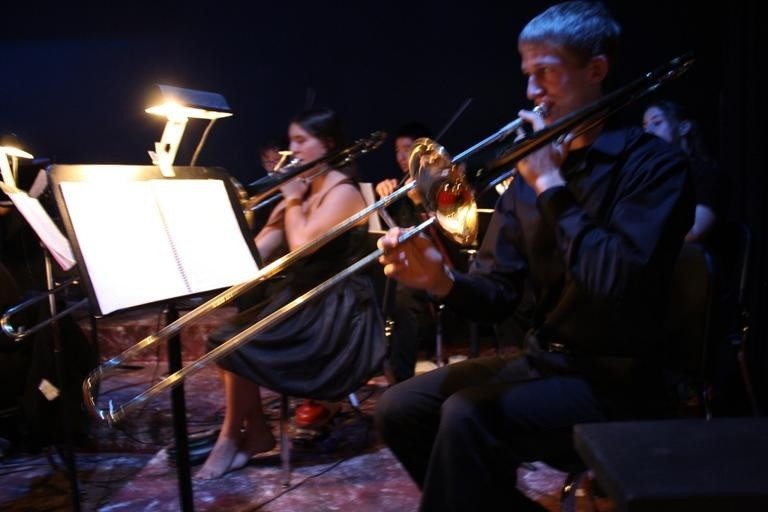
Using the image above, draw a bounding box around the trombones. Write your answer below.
[0,130,388,340]
[82,55,695,427]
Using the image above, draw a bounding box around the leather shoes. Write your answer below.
[167,447,214,468]
[166,429,220,454]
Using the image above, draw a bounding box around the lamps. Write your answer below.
[144,83,233,166]
[0,129,35,208]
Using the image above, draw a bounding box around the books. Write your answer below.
[56,178,261,317]
[7,191,78,273]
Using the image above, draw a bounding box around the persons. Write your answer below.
[194,102,391,483]
[371,127,467,391]
[234,124,310,316]
[637,81,737,244]
[373,1,704,511]
